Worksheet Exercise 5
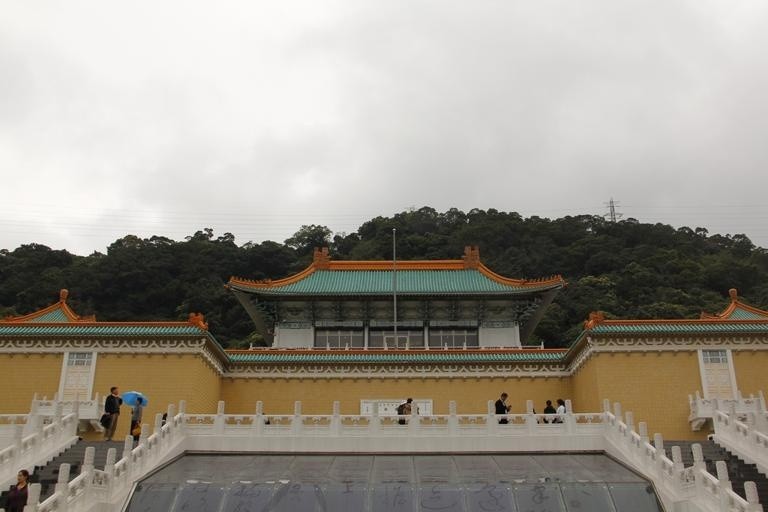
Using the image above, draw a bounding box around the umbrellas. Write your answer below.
[121,390,148,408]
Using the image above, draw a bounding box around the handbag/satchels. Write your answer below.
[131,427,142,436]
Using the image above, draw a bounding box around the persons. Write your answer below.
[555,398,565,423]
[543,399,556,423]
[261,413,271,424]
[160,412,168,427]
[5,469,29,511]
[103,386,123,441]
[495,392,512,424]
[402,398,419,425]
[129,397,142,442]
[397,401,406,425]
